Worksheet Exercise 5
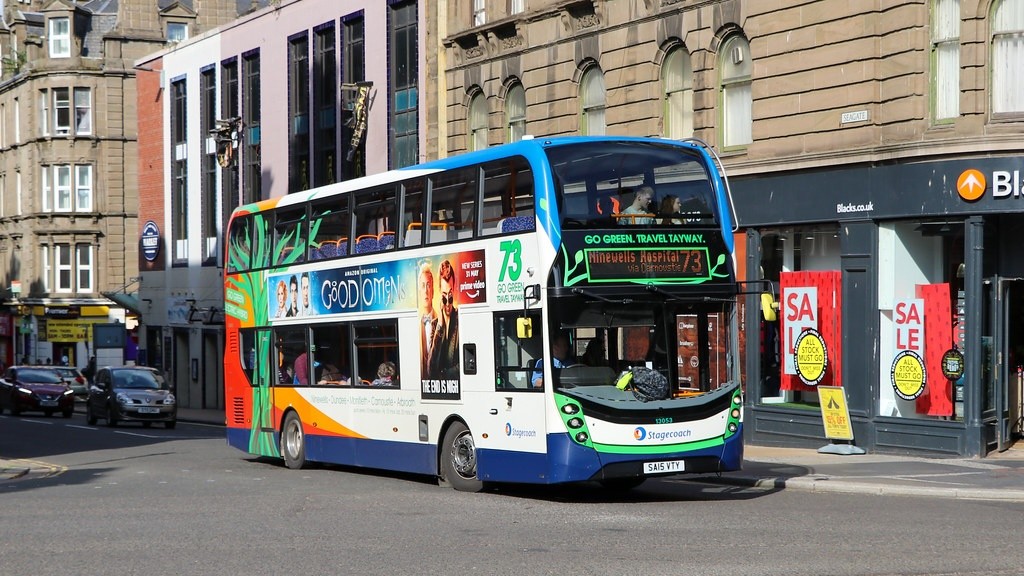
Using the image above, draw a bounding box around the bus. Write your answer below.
[223,134,783,492]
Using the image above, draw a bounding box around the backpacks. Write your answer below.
[630,366,669,403]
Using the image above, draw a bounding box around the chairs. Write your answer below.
[281,247,301,262]
[337,238,356,256]
[377,231,404,251]
[357,235,377,253]
[315,241,337,258]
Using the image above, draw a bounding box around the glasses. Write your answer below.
[443,296,454,305]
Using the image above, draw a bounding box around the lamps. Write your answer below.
[207,116,241,144]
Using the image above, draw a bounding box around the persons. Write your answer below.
[619,184,660,226]
[294,342,399,387]
[285,275,299,317]
[419,260,461,400]
[19,356,66,367]
[274,280,288,318]
[296,272,320,317]
[531,332,582,388]
[653,193,687,225]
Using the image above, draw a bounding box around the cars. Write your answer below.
[85,365,178,430]
[0,365,89,420]
